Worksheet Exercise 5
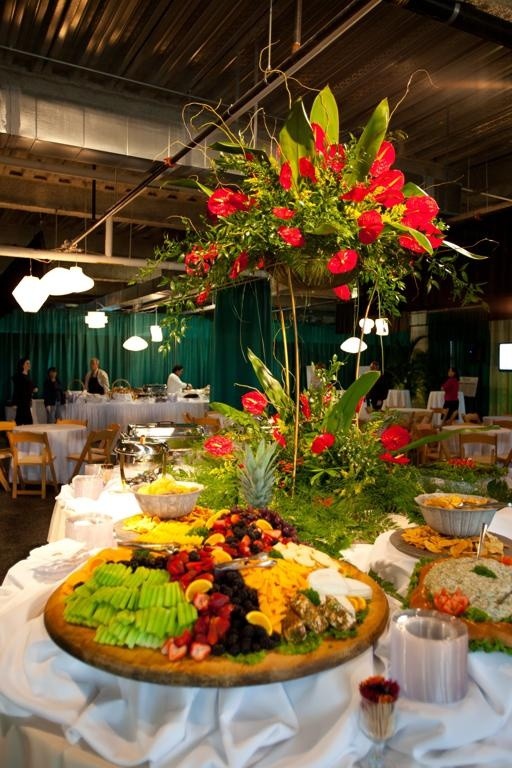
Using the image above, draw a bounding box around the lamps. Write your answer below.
[122,312,148,352]
[340,300,368,353]
[40,213,75,296]
[149,308,163,341]
[85,298,109,329]
[12,258,50,312]
[374,316,389,337]
[358,318,374,335]
[70,260,94,294]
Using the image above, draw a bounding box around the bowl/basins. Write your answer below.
[114,436,168,484]
[414,493,498,536]
[132,481,203,518]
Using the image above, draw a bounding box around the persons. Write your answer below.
[441,367,459,420]
[43,368,65,424]
[167,364,192,396]
[13,359,38,425]
[84,359,110,396]
[368,363,382,414]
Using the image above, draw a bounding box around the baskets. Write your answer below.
[108,379,134,400]
[66,379,85,402]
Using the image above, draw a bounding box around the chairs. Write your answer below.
[492,419,512,430]
[192,417,221,436]
[417,428,450,462]
[0,420,24,492]
[497,448,512,467]
[182,411,192,424]
[89,424,120,453]
[7,430,59,499]
[459,433,498,466]
[56,418,88,427]
[66,431,113,484]
[430,407,448,430]
[462,413,481,425]
[413,412,432,429]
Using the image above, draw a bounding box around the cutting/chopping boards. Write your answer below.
[113,513,142,540]
[43,545,389,688]
[389,527,511,563]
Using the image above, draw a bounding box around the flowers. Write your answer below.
[124,63,487,288]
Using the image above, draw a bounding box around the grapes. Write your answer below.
[219,508,295,554]
